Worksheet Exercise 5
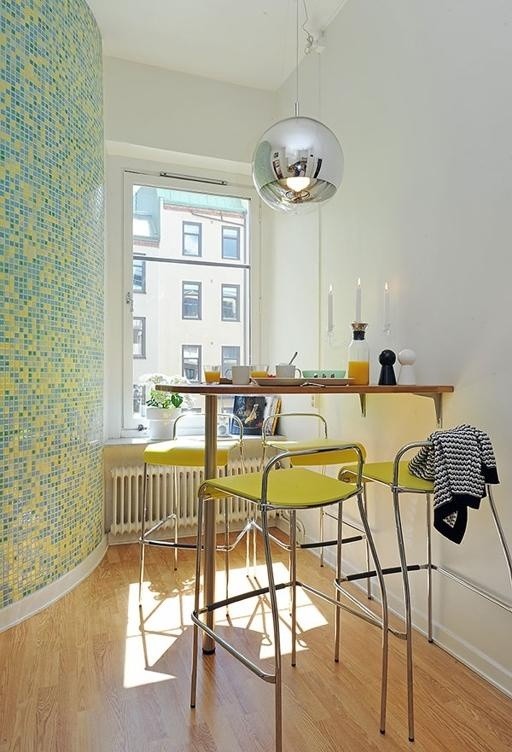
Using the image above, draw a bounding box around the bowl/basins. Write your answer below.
[303,369,346,378]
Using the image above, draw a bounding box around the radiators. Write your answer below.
[105,456,287,538]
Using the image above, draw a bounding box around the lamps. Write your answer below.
[251,1,344,213]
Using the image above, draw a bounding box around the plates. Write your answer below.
[254,377,305,386]
[304,378,355,387]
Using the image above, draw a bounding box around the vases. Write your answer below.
[145,406,184,442]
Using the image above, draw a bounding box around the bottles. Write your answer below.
[347,330,370,384]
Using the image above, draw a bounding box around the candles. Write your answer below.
[383,281,391,324]
[355,278,362,322]
[327,284,333,333]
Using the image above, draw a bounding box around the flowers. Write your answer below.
[136,371,195,411]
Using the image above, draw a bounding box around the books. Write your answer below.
[229,395,281,436]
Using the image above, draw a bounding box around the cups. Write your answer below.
[199,363,302,386]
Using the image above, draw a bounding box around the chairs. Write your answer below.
[257,410,375,615]
[189,441,394,752]
[129,412,246,615]
[341,442,512,742]
